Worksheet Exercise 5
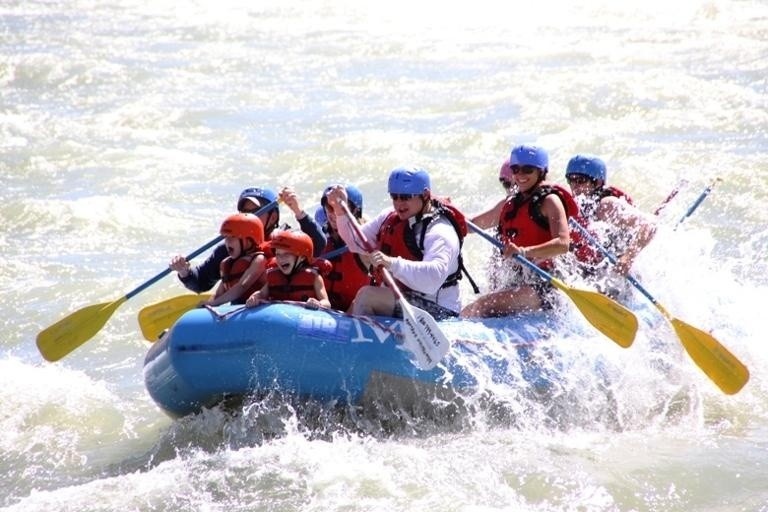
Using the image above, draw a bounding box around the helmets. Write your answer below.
[388,166,431,195]
[510,145,548,168]
[238,188,279,208]
[566,155,606,180]
[271,230,313,256]
[499,161,514,180]
[221,213,264,244]
[321,183,362,208]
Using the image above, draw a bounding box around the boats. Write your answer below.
[143,305,675,417]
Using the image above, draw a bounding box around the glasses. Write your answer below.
[390,193,412,201]
[511,165,534,174]
[566,175,589,184]
[503,182,511,188]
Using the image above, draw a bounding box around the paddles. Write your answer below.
[336,195,450,371]
[36,196,284,363]
[568,216,749,395]
[137,245,349,343]
[465,218,638,348]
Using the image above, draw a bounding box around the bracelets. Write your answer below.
[519,246,526,259]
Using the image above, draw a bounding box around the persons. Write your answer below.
[561,154,656,301]
[441,145,569,319]
[498,158,518,198]
[325,167,460,318]
[168,183,384,314]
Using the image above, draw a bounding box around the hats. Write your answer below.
[238,196,261,208]
